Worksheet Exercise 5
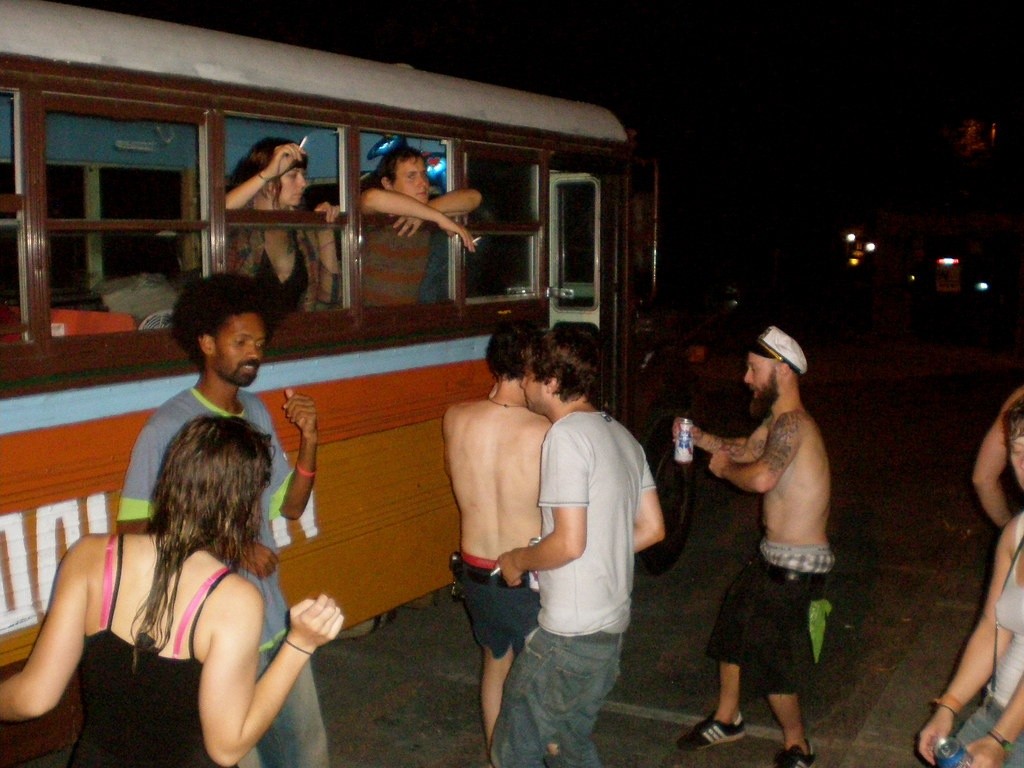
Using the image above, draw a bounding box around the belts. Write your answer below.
[462,565,532,590]
[759,559,827,587]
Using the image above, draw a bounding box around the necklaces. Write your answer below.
[488,396,528,408]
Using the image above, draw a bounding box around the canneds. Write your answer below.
[933,737,973,768]
[674,417,694,463]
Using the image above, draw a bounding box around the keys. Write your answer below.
[451,580,455,595]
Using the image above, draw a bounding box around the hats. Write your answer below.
[742,325,808,375]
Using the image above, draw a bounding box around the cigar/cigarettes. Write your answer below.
[300,135,308,149]
[472,235,482,242]
[490,567,500,576]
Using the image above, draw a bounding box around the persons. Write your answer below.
[671,325,837,768]
[440,318,560,768]
[118,270,330,768]
[0,414,346,768]
[914,384,1024,768]
[103,129,482,333]
[489,322,666,768]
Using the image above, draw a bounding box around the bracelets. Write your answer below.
[295,461,316,477]
[988,727,1012,752]
[319,239,335,250]
[934,692,963,714]
[258,171,270,182]
[283,636,314,657]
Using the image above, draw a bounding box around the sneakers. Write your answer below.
[774,738,814,768]
[677,710,746,752]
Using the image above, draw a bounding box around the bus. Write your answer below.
[1,1,704,768]
